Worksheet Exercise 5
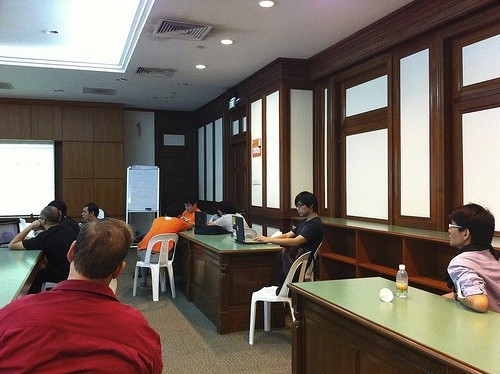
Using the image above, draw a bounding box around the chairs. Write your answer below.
[305,242,322,282]
[249,251,312,346]
[133,233,178,302]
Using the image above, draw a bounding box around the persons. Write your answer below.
[207,201,249,233]
[255,191,324,273]
[8,206,77,295]
[180,194,202,227]
[47,200,81,236]
[137,203,193,289]
[81,203,99,223]
[0,218,164,374]
[442,203,500,312]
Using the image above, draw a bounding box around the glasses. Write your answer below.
[449,224,462,228]
[296,203,304,208]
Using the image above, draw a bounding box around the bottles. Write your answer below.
[395,264,408,298]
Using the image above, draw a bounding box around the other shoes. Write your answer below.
[138,280,146,288]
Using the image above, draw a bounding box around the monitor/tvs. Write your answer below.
[195,211,207,229]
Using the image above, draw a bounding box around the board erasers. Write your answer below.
[145,208,152,211]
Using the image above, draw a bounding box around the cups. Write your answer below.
[379,288,393,302]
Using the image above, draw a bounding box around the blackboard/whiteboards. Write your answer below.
[129,168,158,212]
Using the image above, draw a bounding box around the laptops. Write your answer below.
[232,216,267,245]
[0,222,20,245]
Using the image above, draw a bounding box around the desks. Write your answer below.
[177,227,285,335]
[287,277,500,374]
[0,247,43,309]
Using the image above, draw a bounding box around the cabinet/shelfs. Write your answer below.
[289,217,500,295]
